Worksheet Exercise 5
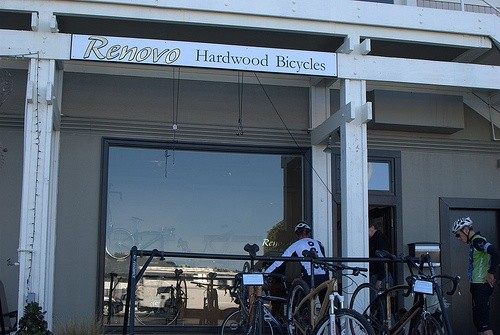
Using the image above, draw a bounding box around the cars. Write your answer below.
[104,295,124,315]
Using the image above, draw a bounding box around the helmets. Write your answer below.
[294,223,311,232]
[452,217,473,234]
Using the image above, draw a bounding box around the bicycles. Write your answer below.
[286,248,374,335]
[347,249,461,335]
[221,295,289,335]
[220,243,275,335]
[166,268,189,324]
[190,271,219,325]
[106,216,175,259]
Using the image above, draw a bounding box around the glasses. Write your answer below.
[456,230,464,237]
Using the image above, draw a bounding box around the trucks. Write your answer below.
[137,265,241,318]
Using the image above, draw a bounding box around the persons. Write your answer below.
[451,216,498,335]
[264,222,330,335]
[369,222,397,334]
